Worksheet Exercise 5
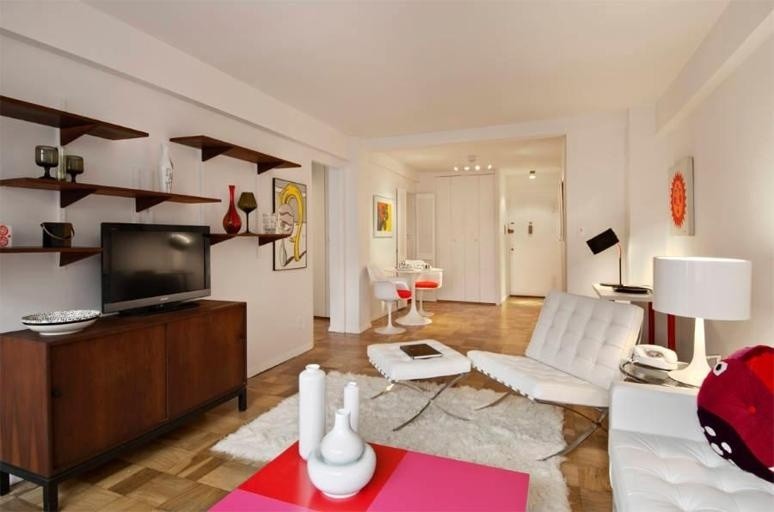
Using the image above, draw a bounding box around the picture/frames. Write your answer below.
[273,178,307,271]
[373,195,393,239]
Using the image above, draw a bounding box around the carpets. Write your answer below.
[212,370,573,512]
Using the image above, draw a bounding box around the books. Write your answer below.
[401,343,442,360]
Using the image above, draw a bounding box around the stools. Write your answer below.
[367,339,470,432]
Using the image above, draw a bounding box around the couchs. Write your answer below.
[608,382,774,512]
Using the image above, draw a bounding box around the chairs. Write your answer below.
[466,291,644,462]
[366,254,444,335]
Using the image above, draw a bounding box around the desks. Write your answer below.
[620,362,699,388]
[207,441,531,512]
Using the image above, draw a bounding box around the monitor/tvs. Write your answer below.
[101,223,211,315]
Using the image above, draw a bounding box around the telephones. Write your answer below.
[633,344,677,371]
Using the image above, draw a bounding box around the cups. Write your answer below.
[425,264,430,269]
[262,213,277,233]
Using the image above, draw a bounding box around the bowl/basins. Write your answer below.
[19,309,102,336]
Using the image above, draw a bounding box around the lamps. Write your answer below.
[586,228,647,294]
[651,258,752,388]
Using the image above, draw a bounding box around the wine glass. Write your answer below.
[35,145,59,180]
[237,191,257,234]
[65,156,83,183]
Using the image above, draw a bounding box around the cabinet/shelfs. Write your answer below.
[0,300,247,512]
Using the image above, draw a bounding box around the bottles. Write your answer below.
[157,143,172,193]
[223,184,242,234]
[298,363,377,500]
